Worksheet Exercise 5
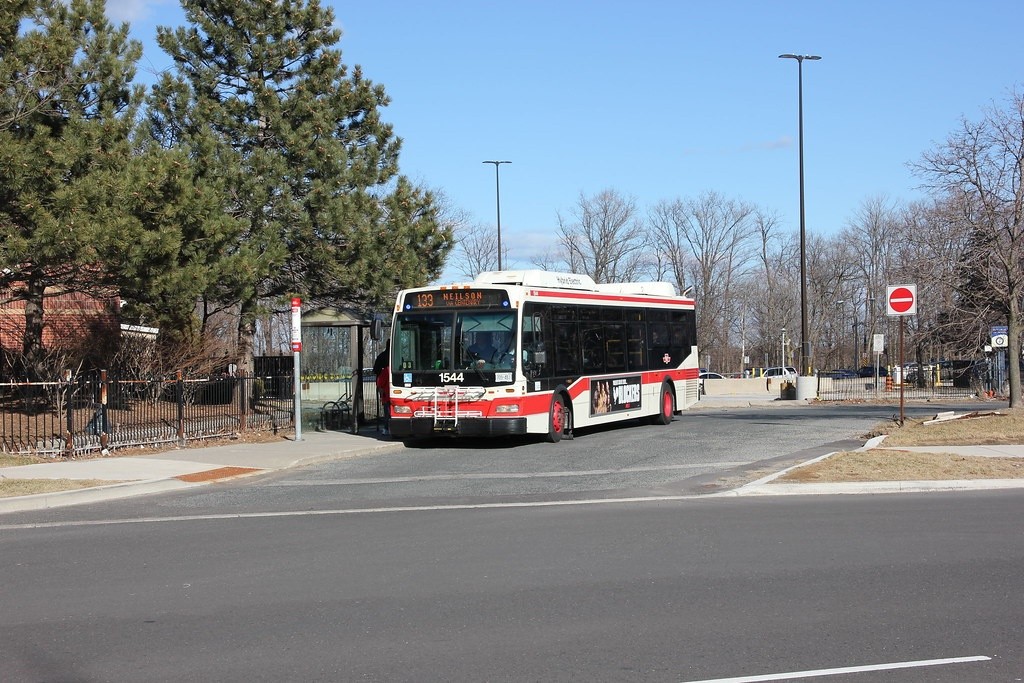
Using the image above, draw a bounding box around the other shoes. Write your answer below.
[381,428,388,435]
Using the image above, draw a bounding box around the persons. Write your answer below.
[593,381,612,414]
[373,339,390,375]
[377,366,390,435]
[467,332,498,361]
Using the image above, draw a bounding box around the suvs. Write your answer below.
[907,359,978,388]
[755,366,799,378]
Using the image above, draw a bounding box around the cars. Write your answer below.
[699,373,727,389]
[362,367,377,381]
[819,365,888,379]
[731,367,768,378]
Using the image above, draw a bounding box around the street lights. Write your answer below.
[781,327,786,376]
[484,160,513,270]
[780,53,821,375]
[838,298,876,370]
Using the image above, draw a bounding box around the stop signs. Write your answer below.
[886,284,917,315]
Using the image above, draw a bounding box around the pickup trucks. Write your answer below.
[896,363,936,383]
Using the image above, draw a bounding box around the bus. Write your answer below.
[387,269,702,443]
